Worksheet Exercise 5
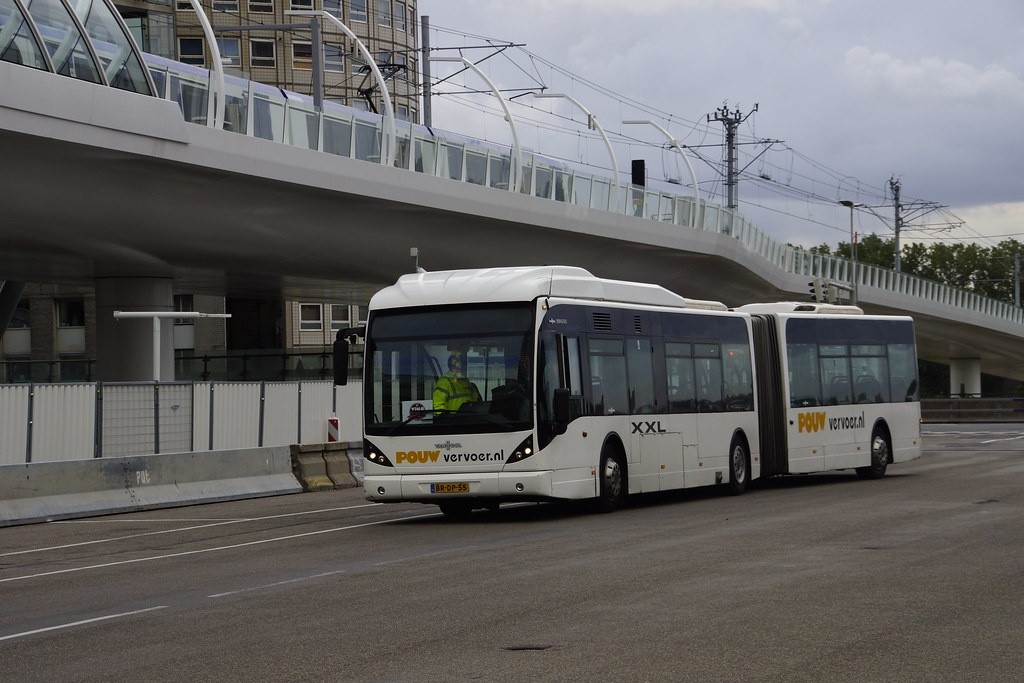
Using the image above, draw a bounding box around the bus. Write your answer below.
[331,264,922,516]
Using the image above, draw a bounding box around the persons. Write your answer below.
[434,354,478,415]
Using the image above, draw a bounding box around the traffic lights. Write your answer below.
[823,281,836,303]
[808,279,823,301]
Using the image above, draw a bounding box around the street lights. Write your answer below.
[535,93,620,212]
[284,10,396,168]
[429,55,523,195]
[840,201,856,304]
[623,120,701,230]
